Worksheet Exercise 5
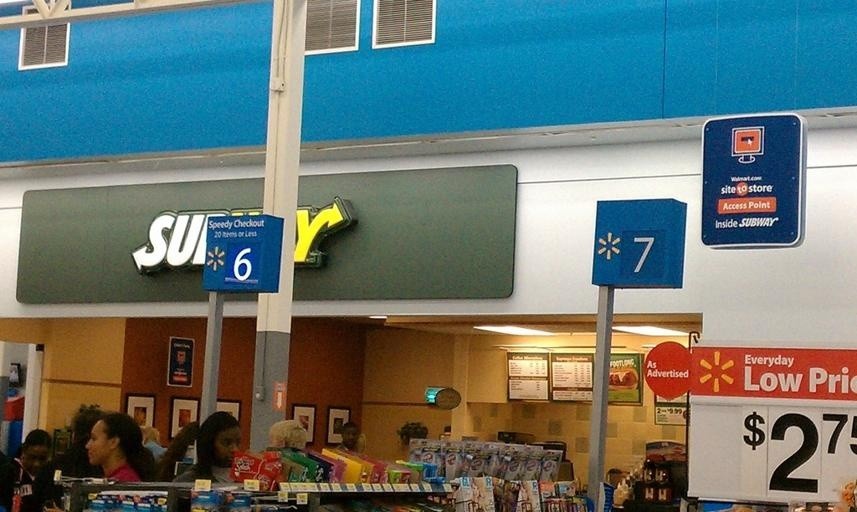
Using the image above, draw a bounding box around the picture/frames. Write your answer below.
[124,393,156,429]
[291,403,316,446]
[216,399,241,422]
[167,396,200,441]
[324,405,351,446]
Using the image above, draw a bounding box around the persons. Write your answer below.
[173,411,242,484]
[0,410,198,512]
[268,420,308,458]
[337,423,359,452]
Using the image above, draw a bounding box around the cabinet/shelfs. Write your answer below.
[69,481,457,511]
[622,461,696,512]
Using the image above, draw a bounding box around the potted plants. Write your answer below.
[396,422,428,461]
